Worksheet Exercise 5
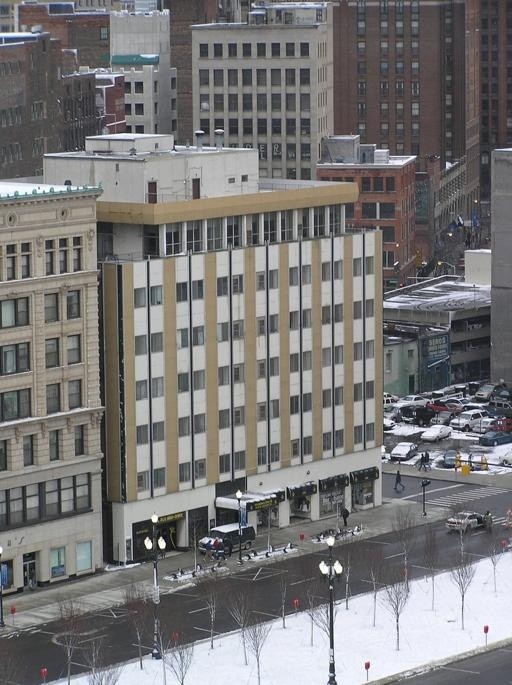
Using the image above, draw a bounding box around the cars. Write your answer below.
[498,450,512,465]
[469,454,489,471]
[445,510,493,533]
[383,384,512,460]
[443,449,459,467]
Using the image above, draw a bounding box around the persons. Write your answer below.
[171,563,201,582]
[483,510,493,534]
[467,450,472,467]
[418,453,427,472]
[424,450,432,470]
[392,470,405,490]
[339,505,350,527]
[202,536,233,565]
[455,451,463,471]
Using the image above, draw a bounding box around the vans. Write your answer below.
[198,523,256,555]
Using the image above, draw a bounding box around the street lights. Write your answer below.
[144,514,166,659]
[319,535,342,685]
[235,488,243,565]
[0,547,5,626]
